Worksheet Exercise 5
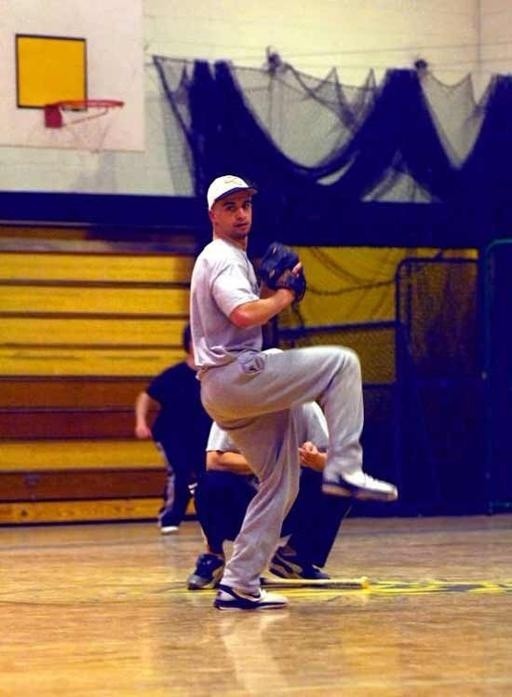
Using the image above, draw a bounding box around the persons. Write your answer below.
[184,345,356,588]
[188,174,398,613]
[134,324,219,535]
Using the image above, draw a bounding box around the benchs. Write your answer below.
[0,220,196,524]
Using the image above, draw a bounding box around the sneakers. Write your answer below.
[187,554,226,590]
[213,584,288,611]
[269,546,330,579]
[160,526,179,534]
[321,465,398,502]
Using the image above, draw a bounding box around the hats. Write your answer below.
[207,175,258,212]
